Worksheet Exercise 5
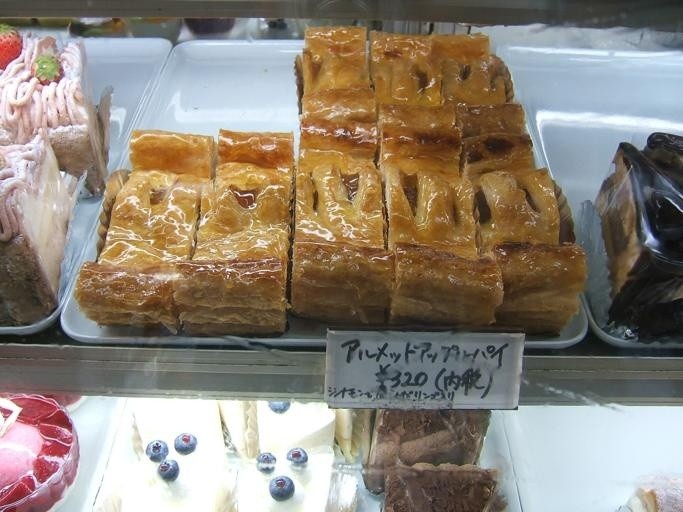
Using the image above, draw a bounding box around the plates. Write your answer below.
[0,30,178,337]
[87,394,524,512]
[57,39,591,349]
[1,392,124,512]
[502,409,683,512]
[493,44,683,353]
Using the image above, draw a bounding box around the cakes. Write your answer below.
[360,402,489,489]
[93,397,336,512]
[644,131,683,186]
[0,393,80,512]
[337,406,370,460]
[1,28,109,198]
[597,143,683,334]
[381,462,500,512]
[0,125,71,327]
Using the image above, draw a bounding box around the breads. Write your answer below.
[302,26,376,121]
[371,30,442,113]
[457,103,587,338]
[380,103,504,327]
[171,128,293,337]
[75,132,211,329]
[293,116,392,323]
[437,35,511,105]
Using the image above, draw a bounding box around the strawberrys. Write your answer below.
[31,54,63,84]
[0,24,23,71]
